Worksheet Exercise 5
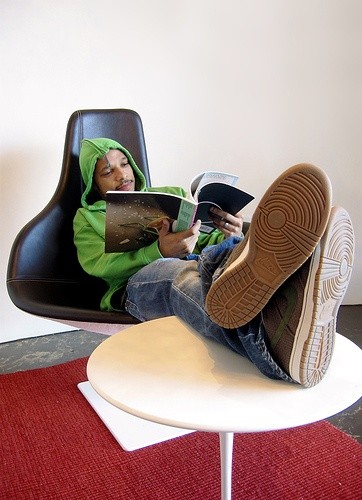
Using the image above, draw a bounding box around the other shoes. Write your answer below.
[260,205,354,388]
[205,163,332,330]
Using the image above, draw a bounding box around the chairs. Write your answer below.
[7,109,199,451]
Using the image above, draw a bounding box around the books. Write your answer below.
[104,171,255,254]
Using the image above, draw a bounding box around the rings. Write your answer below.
[224,222,229,230]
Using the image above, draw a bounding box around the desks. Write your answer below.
[85,314,362,500]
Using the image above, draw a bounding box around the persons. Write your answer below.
[73,138,354,387]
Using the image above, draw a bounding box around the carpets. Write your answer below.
[0,356,362,500]
